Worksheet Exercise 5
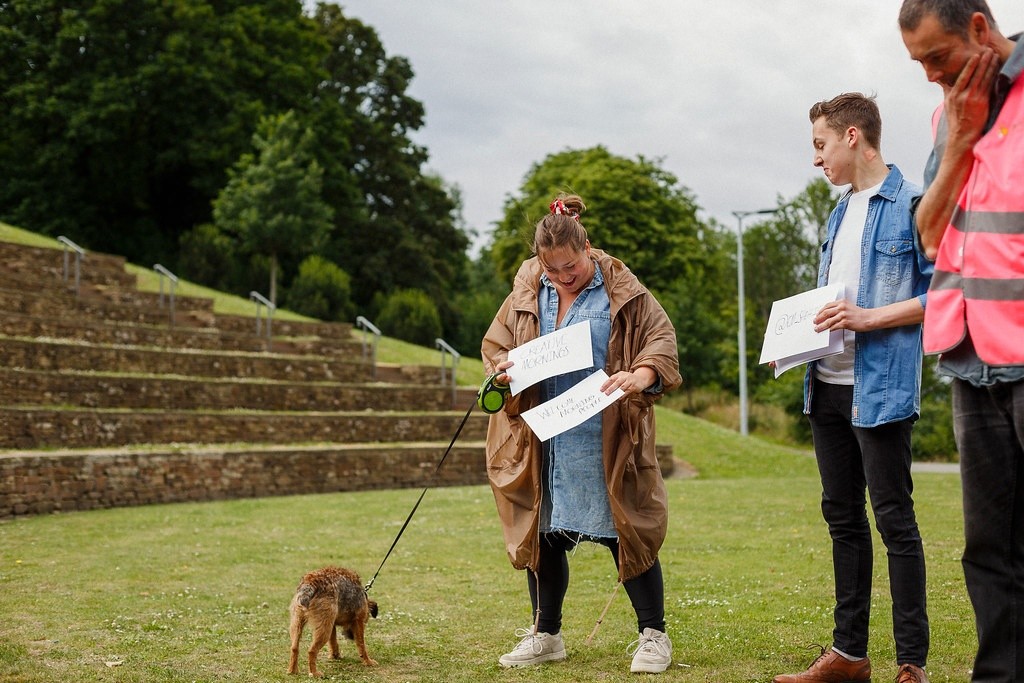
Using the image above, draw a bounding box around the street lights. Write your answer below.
[731,208,778,436]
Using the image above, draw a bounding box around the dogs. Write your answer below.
[285,565,379,679]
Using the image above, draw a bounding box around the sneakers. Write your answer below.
[626,627,672,674]
[498,625,566,668]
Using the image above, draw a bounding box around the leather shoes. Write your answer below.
[895,663,929,683]
[772,643,871,683]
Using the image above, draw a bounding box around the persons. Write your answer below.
[481,192,682,673]
[897,0,1024,683]
[769,91,934,683]
